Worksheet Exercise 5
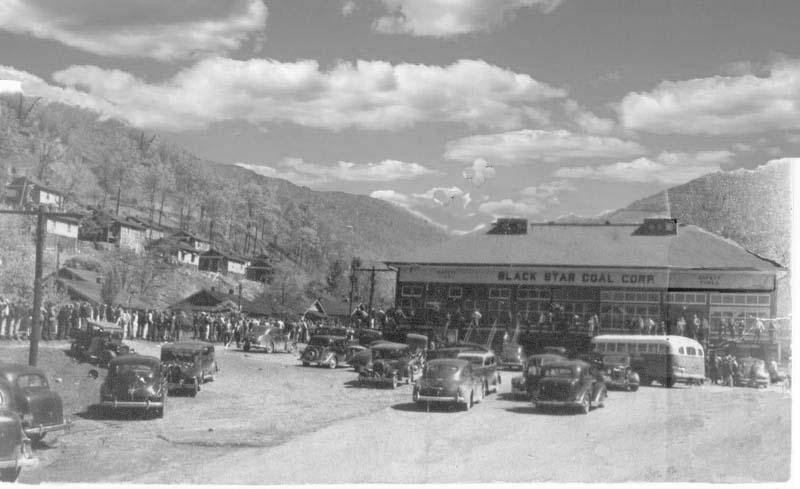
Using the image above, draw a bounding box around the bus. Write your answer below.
[590,334,705,388]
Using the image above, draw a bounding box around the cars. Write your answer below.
[734,357,770,387]
[0,362,65,483]
[71,319,218,419]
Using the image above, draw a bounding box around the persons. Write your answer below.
[352,303,482,335]
[190,310,344,342]
[536,307,710,339]
[0,294,184,343]
[701,312,790,388]
